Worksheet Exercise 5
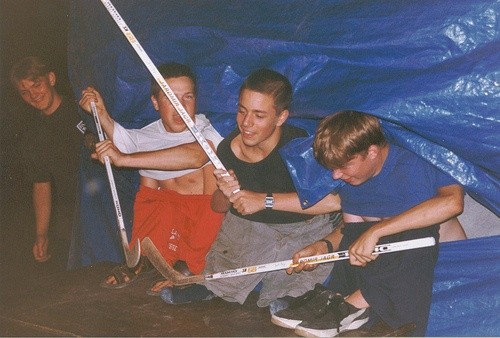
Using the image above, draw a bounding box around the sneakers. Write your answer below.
[294,291,375,338]
[271,282,343,330]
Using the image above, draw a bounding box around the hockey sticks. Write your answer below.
[86,85,140,268]
[142,235,436,286]
[100,0,241,193]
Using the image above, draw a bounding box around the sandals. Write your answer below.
[101,253,160,290]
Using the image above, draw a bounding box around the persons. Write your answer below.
[79,63,226,296]
[211,69,341,308]
[271,110,464,338]
[10,57,86,263]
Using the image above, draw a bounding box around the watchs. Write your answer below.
[266,192,273,210]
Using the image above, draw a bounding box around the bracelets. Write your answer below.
[320,240,333,253]
